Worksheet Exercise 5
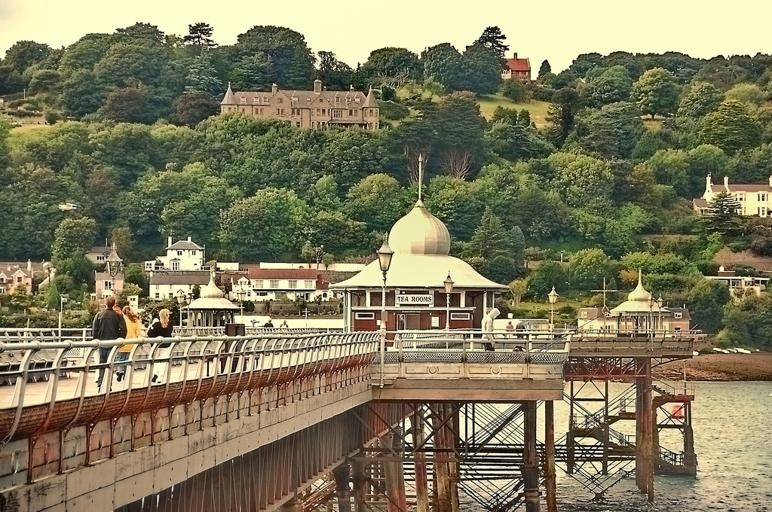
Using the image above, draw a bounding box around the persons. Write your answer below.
[92,297,127,393]
[506,322,514,338]
[114,305,140,381]
[147,309,173,383]
[481,308,501,351]
[516,321,526,338]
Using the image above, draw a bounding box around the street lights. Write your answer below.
[546,283,561,349]
[103,239,124,298]
[374,230,394,331]
[236,281,247,325]
[442,270,457,351]
[175,288,187,332]
[646,290,665,343]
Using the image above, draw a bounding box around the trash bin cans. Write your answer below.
[226,323,245,353]
